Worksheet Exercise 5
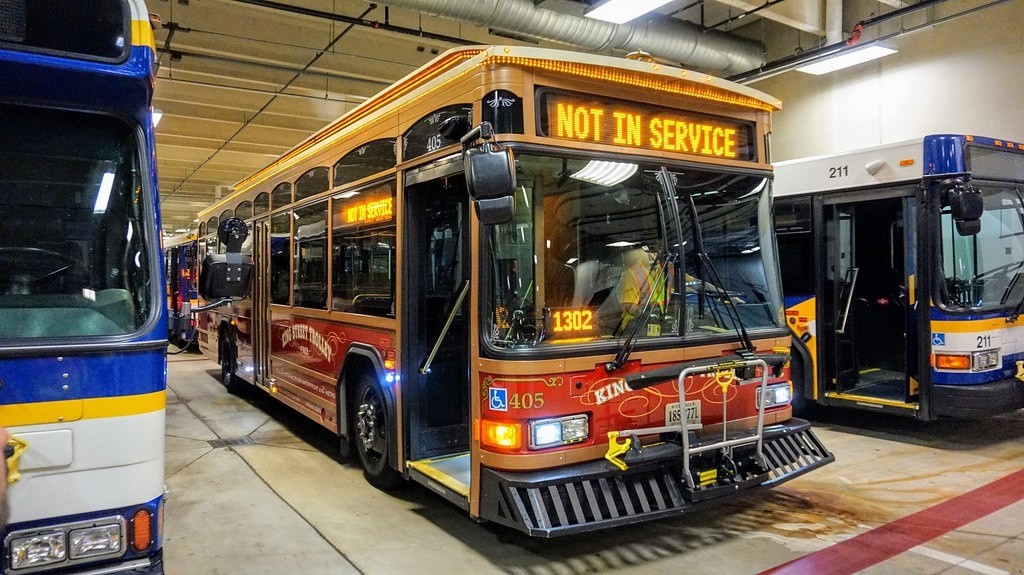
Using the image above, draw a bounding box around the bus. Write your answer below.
[163,230,204,349]
[0,0,255,575]
[195,45,835,540]
[761,134,1024,423]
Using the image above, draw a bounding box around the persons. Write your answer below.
[618,235,746,334]
